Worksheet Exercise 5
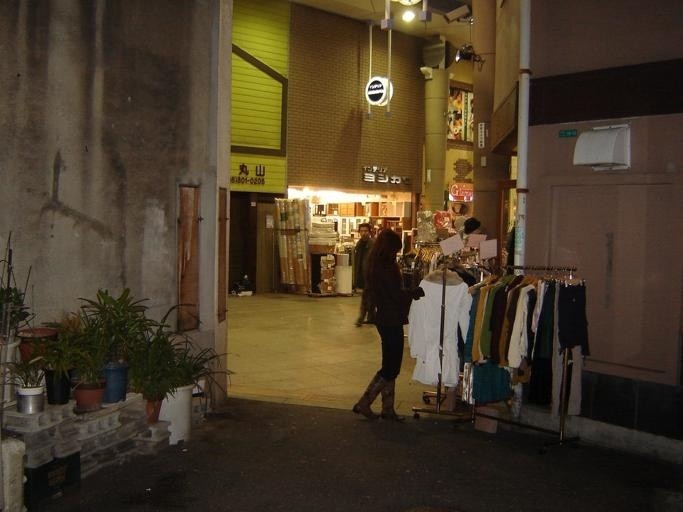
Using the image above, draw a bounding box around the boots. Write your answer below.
[352,371,387,418]
[381,379,405,420]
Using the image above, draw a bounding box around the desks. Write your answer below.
[308,244,336,293]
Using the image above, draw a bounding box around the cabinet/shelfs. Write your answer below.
[314,201,412,237]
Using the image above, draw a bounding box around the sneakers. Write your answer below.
[363,320,373,324]
[354,321,362,325]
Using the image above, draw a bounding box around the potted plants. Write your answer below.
[0,287,236,446]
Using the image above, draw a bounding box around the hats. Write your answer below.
[464,218,480,234]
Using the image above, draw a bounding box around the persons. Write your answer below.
[350,223,381,327]
[352,224,405,422]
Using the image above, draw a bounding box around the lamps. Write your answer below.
[421,67,432,79]
[366,18,394,118]
[573,123,631,171]
[455,45,479,63]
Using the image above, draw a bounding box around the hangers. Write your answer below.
[417,241,583,286]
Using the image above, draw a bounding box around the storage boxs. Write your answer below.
[320,256,335,293]
